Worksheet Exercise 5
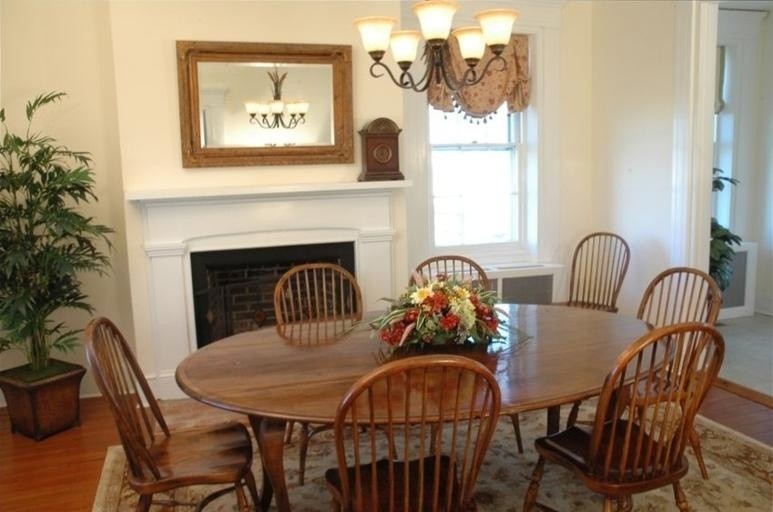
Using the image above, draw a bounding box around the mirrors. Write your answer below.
[176,40,354,168]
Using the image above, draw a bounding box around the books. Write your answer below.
[541,424,608,469]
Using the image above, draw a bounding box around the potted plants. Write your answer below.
[0,92,118,441]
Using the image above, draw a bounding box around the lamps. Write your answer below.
[356,0,520,92]
[244,67,309,129]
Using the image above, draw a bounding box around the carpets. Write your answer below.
[93,397,773,512]
[704,311,772,398]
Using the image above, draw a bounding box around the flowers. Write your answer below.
[344,272,532,350]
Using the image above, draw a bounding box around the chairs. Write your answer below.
[567,268,722,482]
[409,255,522,456]
[521,323,726,512]
[84,315,258,512]
[567,231,630,311]
[274,262,395,486]
[323,353,501,511]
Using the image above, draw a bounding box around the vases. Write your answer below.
[395,342,491,353]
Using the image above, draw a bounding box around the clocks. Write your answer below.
[358,118,404,182]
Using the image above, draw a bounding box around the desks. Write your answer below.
[175,302,680,512]
